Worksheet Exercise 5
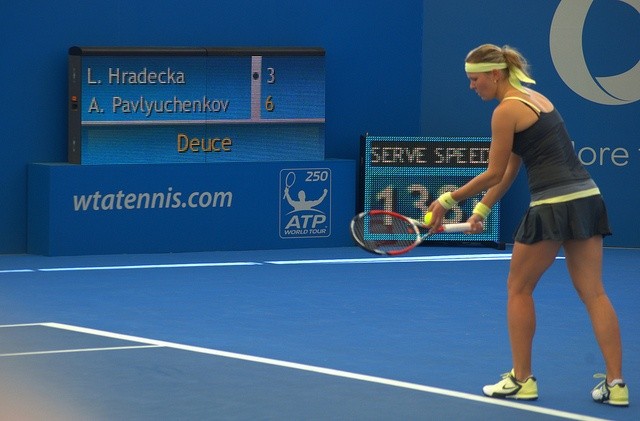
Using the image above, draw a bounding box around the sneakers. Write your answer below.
[591,373,629,405]
[483,369,539,400]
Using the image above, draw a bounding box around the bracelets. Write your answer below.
[437,192,457,210]
[472,202,492,220]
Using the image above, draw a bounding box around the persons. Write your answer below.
[422,43,630,407]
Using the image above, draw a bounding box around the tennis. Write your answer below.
[424,212,433,224]
